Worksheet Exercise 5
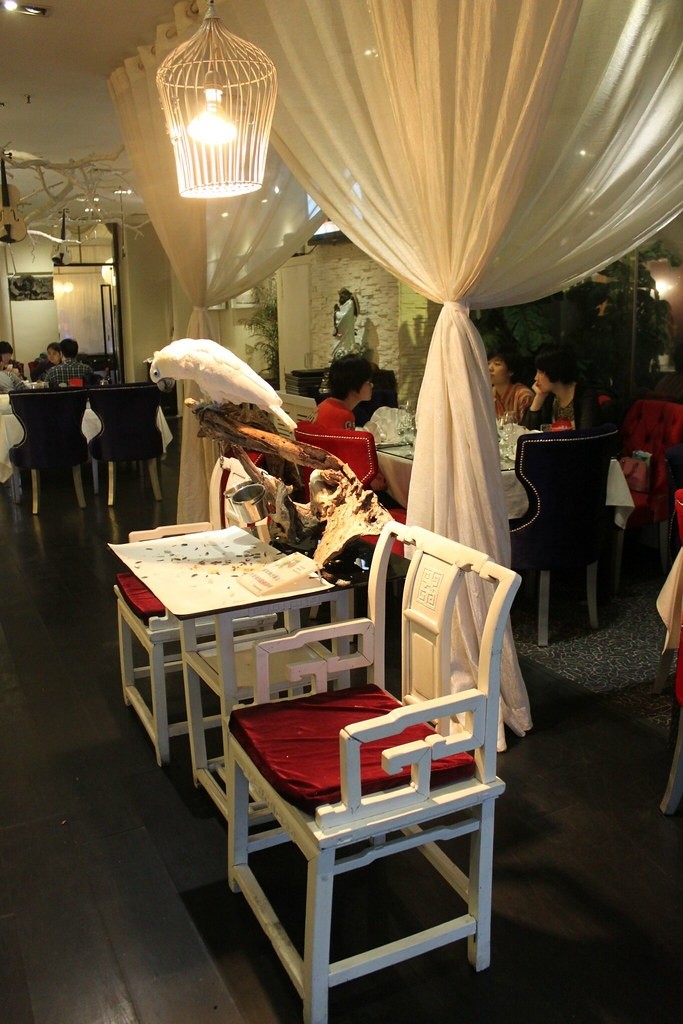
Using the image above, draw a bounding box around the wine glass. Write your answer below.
[397,400,417,456]
[497,411,519,459]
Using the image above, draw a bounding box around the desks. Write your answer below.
[382,422,634,591]
[0,390,174,504]
[144,522,412,826]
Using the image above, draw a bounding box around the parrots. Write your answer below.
[149,338,298,432]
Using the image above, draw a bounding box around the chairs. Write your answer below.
[506,426,618,647]
[89,385,164,506]
[10,390,89,514]
[216,519,524,1023]
[292,422,378,503]
[613,397,683,593]
[112,455,282,768]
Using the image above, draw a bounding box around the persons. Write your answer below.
[653,342,683,401]
[0,338,93,393]
[303,352,397,647]
[488,341,605,642]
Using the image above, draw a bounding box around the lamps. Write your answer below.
[155,1,279,199]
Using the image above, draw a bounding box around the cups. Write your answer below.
[223,480,269,523]
[540,424,552,432]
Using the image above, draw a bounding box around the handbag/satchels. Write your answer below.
[618,456,651,492]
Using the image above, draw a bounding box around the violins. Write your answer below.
[0,151,27,243]
